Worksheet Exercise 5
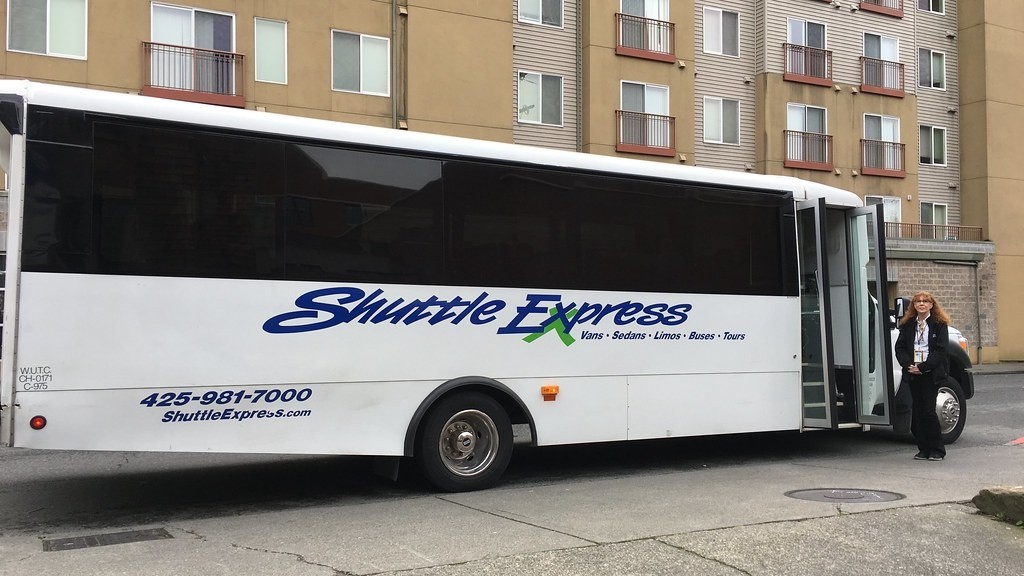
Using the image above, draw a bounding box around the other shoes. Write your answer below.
[928,451,943,461]
[914,452,929,460]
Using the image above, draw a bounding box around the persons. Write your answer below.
[895,292,952,461]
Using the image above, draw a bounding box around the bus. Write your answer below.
[0,74,975,492]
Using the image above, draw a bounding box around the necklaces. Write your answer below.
[920,325,924,331]
